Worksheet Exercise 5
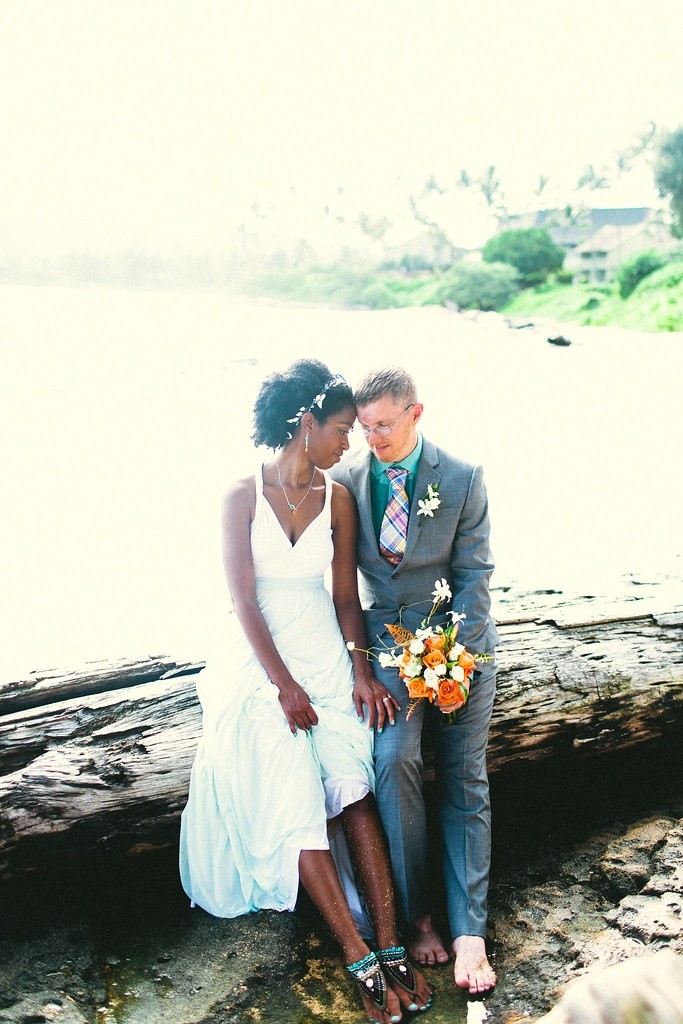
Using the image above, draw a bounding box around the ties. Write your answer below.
[380,469,411,566]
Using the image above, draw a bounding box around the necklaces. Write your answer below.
[273,458,317,515]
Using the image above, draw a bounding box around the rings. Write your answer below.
[382,697,390,702]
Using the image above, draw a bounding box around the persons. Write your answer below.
[177,356,433,1024]
[314,365,502,994]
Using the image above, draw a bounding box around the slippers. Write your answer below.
[376,946,422,1014]
[346,952,392,1016]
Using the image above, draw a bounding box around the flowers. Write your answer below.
[346,578,498,723]
[416,481,445,517]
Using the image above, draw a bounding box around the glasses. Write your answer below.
[358,403,414,436]
